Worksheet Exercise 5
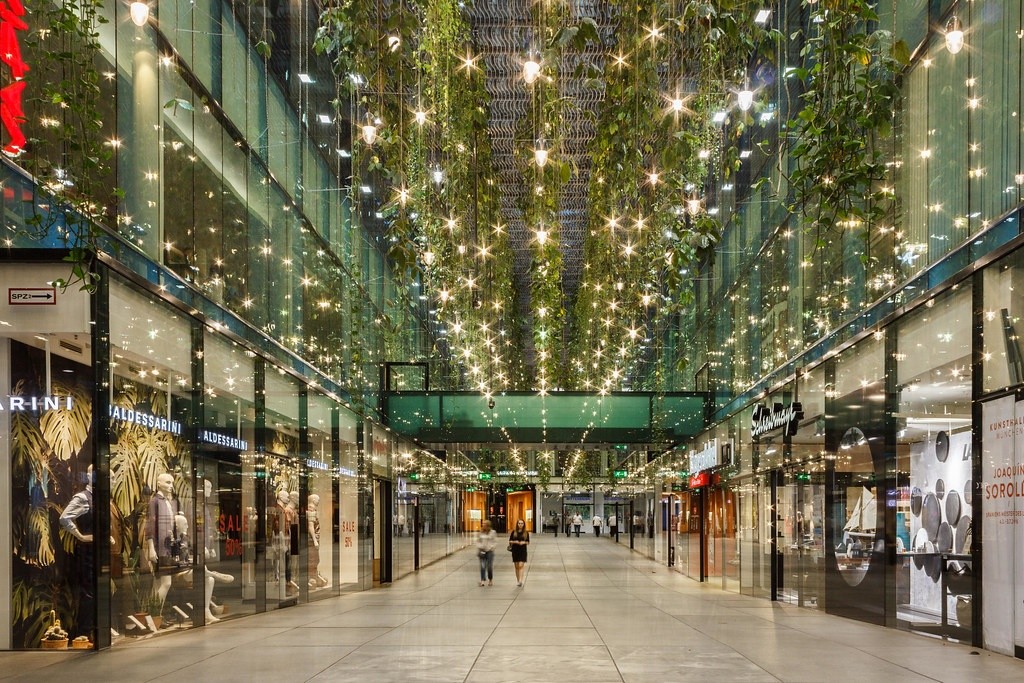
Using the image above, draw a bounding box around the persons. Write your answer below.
[60,463,235,648]
[269,490,326,594]
[477,520,497,587]
[392,512,426,538]
[553,511,602,538]
[606,512,655,537]
[509,519,530,585]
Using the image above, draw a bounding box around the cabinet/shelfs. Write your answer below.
[777,503,793,538]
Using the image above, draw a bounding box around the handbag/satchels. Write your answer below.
[478,550,487,558]
[507,545,512,551]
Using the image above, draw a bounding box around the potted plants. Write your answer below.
[41,610,69,649]
[134,583,164,630]
[72,636,89,648]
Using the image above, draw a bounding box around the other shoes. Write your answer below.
[481,581,485,586]
[488,579,492,586]
[517,581,521,586]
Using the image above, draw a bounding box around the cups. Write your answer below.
[902,548,906,553]
